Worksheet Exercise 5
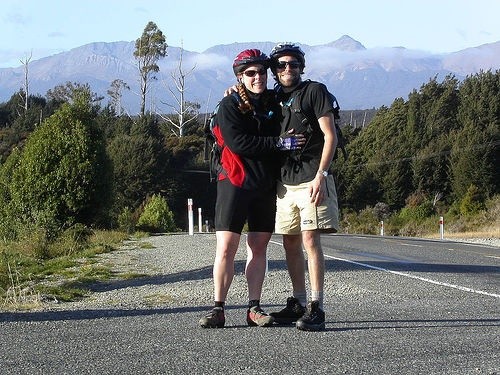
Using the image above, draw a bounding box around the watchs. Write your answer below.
[317,168,328,177]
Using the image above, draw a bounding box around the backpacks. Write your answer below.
[205,104,277,184]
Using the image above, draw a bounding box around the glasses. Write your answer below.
[275,61,301,69]
[239,69,267,76]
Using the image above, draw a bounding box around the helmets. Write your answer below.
[232,49,270,74]
[270,42,305,63]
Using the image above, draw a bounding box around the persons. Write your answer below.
[199,49,307,328]
[223,41,338,331]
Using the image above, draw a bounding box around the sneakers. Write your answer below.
[247,306,274,326]
[200,307,226,328]
[295,301,325,331]
[269,296,308,325]
